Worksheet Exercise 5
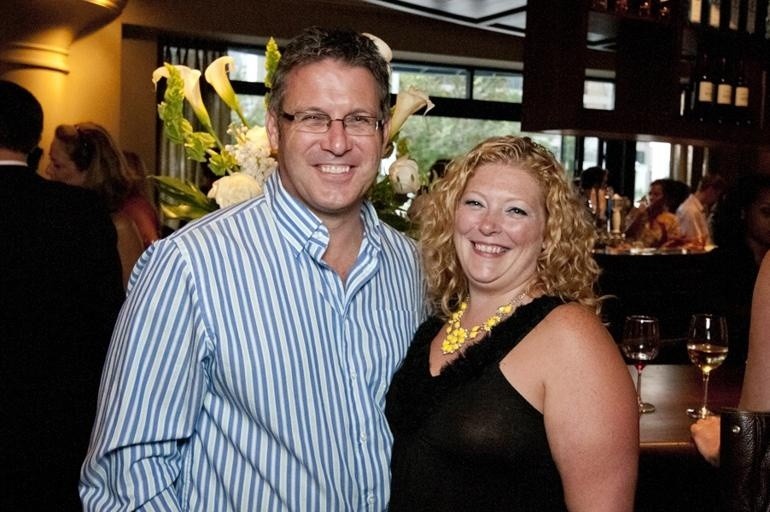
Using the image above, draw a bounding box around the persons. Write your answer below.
[78,21,426,512]
[416,157,451,197]
[691,251,770,469]
[622,173,726,248]
[576,166,607,201]
[119,150,163,247]
[686,170,769,363]
[0,74,125,512]
[384,135,641,512]
[45,120,145,292]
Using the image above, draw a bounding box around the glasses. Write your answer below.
[277,108,384,135]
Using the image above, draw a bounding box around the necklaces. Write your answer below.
[440,291,526,355]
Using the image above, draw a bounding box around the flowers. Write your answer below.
[152,32,433,234]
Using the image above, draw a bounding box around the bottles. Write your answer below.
[695,53,752,126]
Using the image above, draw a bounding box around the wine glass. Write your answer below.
[685,313,729,418]
[619,313,659,413]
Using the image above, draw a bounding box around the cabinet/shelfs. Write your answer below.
[520,0,769,148]
